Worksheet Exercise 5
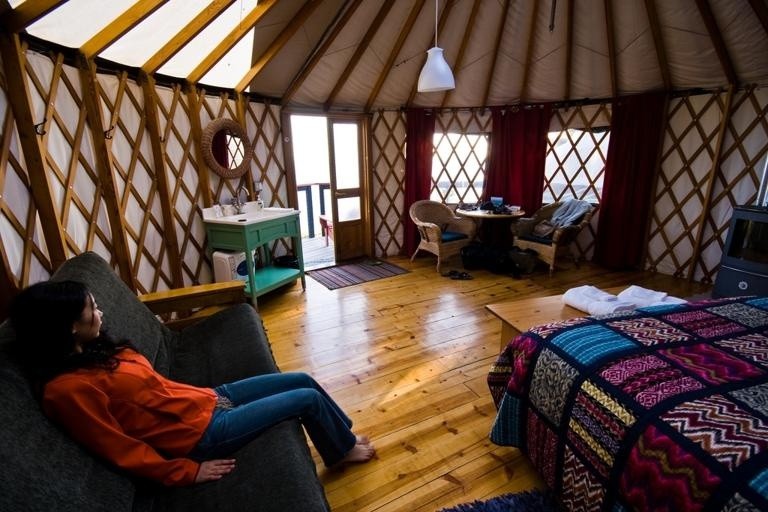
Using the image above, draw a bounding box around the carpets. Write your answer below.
[305,256,413,291]
[437,485,569,511]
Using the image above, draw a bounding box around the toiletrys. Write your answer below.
[254,195,264,211]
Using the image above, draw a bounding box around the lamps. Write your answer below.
[416,1,456,94]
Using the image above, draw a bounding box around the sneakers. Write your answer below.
[442,270,473,280]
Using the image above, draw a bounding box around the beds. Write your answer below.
[486,296,768,511]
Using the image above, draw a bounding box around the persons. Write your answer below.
[11,279,378,489]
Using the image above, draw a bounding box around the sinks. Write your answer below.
[215,207,295,224]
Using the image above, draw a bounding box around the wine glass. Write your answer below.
[491,196,503,214]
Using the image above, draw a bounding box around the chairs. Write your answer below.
[408,198,600,280]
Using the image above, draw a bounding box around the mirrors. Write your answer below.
[202,116,256,181]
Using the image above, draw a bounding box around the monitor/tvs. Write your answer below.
[720,210,768,277]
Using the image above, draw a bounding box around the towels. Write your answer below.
[562,284,688,315]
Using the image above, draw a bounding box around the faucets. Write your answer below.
[230,185,250,212]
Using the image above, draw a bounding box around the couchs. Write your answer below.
[1,250,336,512]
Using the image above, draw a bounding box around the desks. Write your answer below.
[485,284,630,358]
